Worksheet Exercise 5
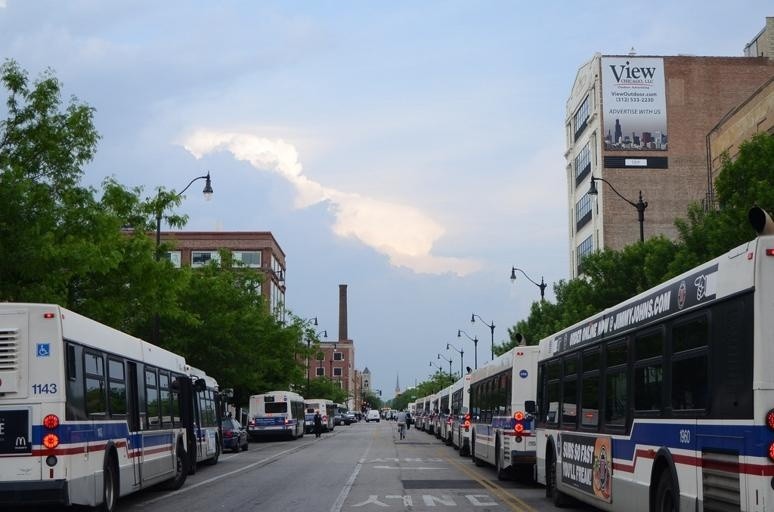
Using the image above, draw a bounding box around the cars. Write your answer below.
[222,418,248,453]
[335,410,361,424]
[380,407,398,420]
[366,410,379,422]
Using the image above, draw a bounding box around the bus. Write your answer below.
[302,398,337,432]
[525,235,774,512]
[247,391,305,441]
[408,334,538,480]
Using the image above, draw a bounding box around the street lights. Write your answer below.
[512,268,546,306]
[472,314,494,361]
[457,330,478,370]
[154,171,212,344]
[588,173,647,242]
[294,317,327,397]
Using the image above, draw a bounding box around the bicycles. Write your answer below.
[397,424,404,440]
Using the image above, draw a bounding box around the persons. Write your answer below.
[404,411,410,429]
[396,408,407,440]
[313,409,322,438]
